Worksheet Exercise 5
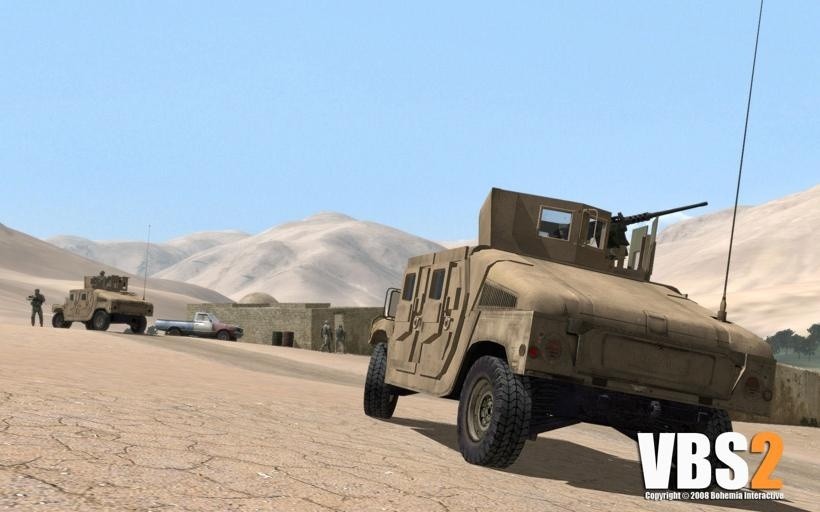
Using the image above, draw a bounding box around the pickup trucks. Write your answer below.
[155,311,244,343]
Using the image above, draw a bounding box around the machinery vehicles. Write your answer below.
[359,0,779,492]
[50,223,156,337]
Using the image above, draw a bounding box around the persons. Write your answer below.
[319,320,334,353]
[335,324,346,354]
[26,289,45,327]
